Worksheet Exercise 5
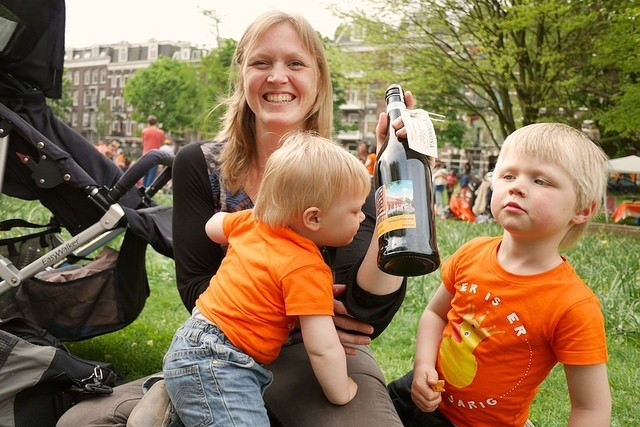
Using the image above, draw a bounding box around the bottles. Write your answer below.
[374,83,441,277]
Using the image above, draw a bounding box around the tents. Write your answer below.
[606,154,640,174]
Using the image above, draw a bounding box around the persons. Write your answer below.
[94,114,173,194]
[126,129,371,427]
[352,141,377,183]
[57,10,435,427]
[387,122,611,427]
[432,158,494,223]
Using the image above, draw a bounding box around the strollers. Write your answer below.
[0,0,173,355]
